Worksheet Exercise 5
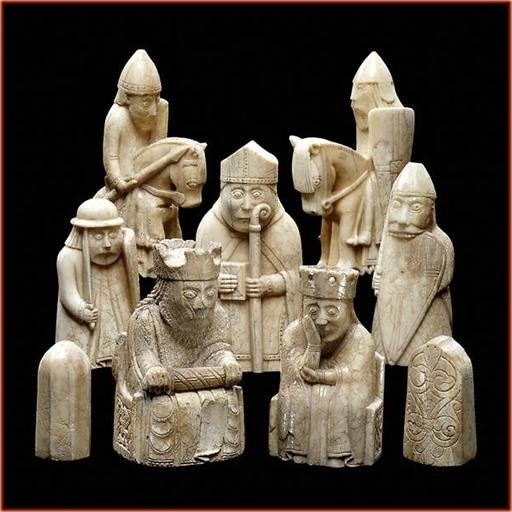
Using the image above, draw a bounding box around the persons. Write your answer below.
[54,197,139,371]
[349,50,414,247]
[277,264,376,467]
[197,137,304,375]
[102,45,171,279]
[370,159,456,368]
[122,236,243,462]
